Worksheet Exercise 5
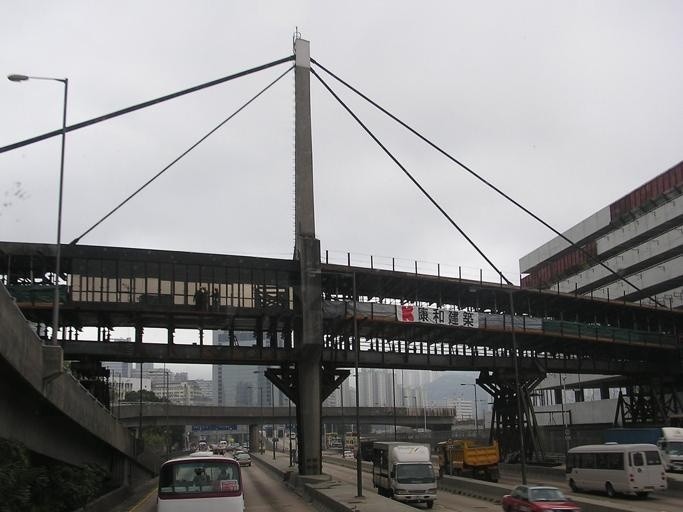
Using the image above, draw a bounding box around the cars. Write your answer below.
[503,485,582,512]
[198,440,251,466]
[291,433,296,439]
[343,450,354,460]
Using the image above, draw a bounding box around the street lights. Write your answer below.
[460,383,478,432]
[248,386,264,454]
[404,396,419,434]
[6,73,70,343]
[562,376,568,456]
[306,267,367,500]
[253,370,277,460]
[149,370,169,462]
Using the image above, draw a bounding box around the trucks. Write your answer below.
[372,441,439,509]
[434,439,500,484]
[657,424,683,473]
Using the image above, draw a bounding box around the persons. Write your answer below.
[192,284,222,307]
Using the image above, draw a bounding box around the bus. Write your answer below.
[155,456,247,512]
[566,440,667,498]
[323,430,357,448]
